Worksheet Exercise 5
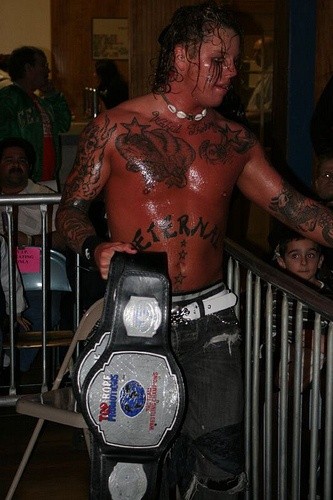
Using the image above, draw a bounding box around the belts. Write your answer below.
[170,287,237,327]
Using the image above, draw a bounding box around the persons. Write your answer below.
[55,6,333,500]
[0,46,129,330]
[271,75,333,393]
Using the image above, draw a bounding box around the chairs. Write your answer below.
[0,248,78,383]
[5,297,105,500]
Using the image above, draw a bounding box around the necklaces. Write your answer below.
[159,87,207,121]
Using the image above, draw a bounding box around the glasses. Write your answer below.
[317,170,333,179]
